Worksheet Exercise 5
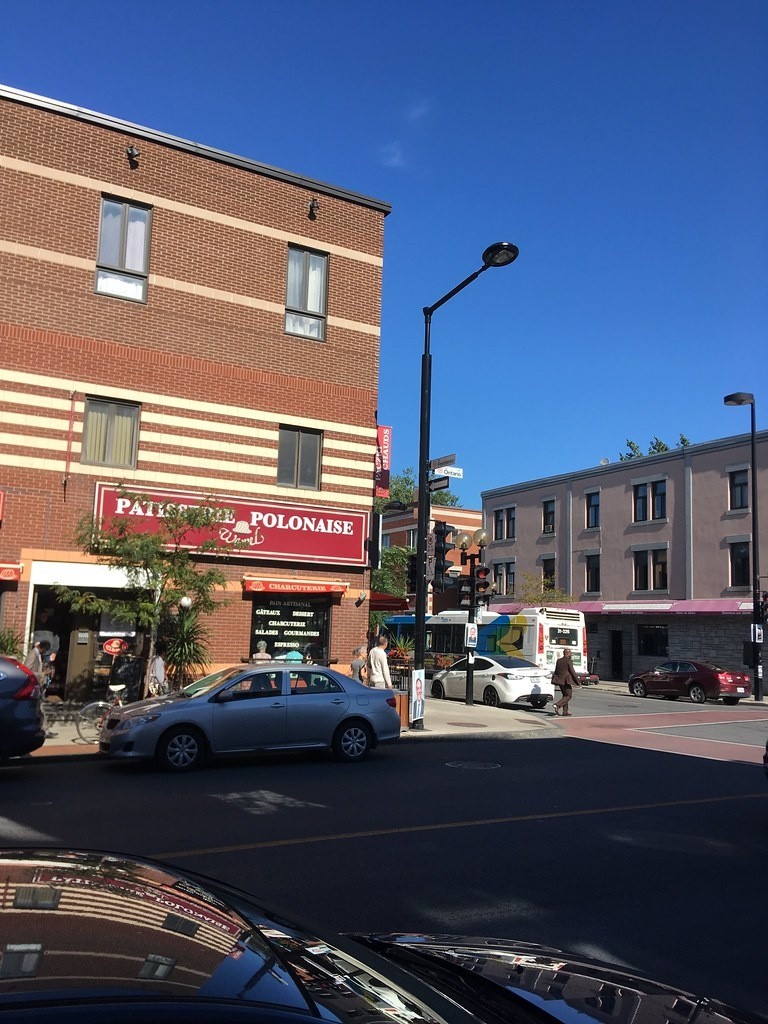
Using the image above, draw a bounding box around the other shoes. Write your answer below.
[553,703,560,716]
[563,712,572,717]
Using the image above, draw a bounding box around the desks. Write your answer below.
[389,666,414,690]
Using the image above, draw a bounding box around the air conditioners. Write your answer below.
[545,525,553,532]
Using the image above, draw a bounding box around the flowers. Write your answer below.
[386,632,415,659]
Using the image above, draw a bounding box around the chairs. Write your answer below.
[250,674,272,690]
[274,675,282,688]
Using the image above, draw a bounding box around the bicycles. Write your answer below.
[74,673,169,746]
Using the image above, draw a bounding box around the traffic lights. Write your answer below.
[761,592,768,626]
[473,566,490,607]
[433,520,456,596]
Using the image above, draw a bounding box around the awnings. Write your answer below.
[0,560,24,581]
[242,576,350,593]
[370,593,409,610]
[488,599,767,615]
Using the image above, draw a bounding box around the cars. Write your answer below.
[627,658,752,705]
[0,843,768,1024]
[0,656,47,760]
[98,658,402,784]
[430,655,555,709]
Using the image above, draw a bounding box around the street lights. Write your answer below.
[409,241,521,730]
[724,392,763,701]
[454,528,492,706]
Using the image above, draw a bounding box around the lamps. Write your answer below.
[126,146,140,160]
[309,199,319,210]
[359,569,367,601]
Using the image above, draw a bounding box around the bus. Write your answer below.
[424,605,590,682]
[379,612,434,655]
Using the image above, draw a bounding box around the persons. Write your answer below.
[253,641,272,659]
[367,637,392,689]
[150,650,169,697]
[24,640,56,736]
[413,678,425,719]
[551,649,580,716]
[348,646,367,683]
[284,640,303,664]
[273,647,286,659]
[469,628,476,643]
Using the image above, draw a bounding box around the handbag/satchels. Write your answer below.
[148,683,159,697]
